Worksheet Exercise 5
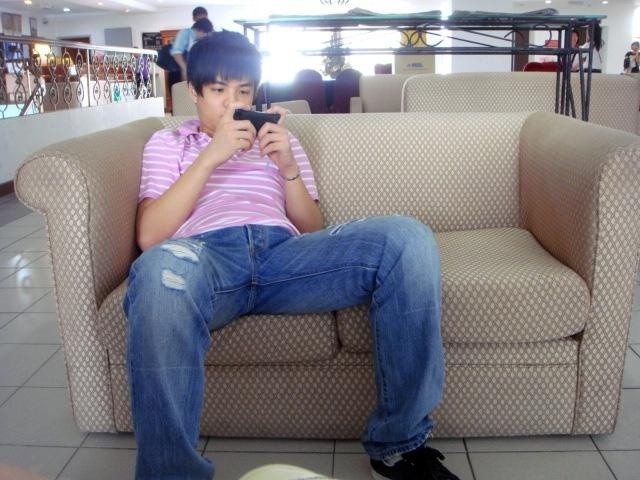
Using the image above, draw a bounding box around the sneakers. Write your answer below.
[368,447,466,480]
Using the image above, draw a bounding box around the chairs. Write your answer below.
[524,61,562,71]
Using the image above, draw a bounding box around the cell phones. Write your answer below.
[234,108,280,136]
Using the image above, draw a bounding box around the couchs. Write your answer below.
[169,76,638,130]
[14,110,637,438]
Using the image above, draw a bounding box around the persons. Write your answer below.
[133,7,218,101]
[123,32,461,480]
[571,22,640,73]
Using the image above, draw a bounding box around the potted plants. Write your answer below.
[39,72,140,108]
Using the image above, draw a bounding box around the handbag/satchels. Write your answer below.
[153,42,189,74]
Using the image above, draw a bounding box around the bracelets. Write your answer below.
[278,166,301,182]
